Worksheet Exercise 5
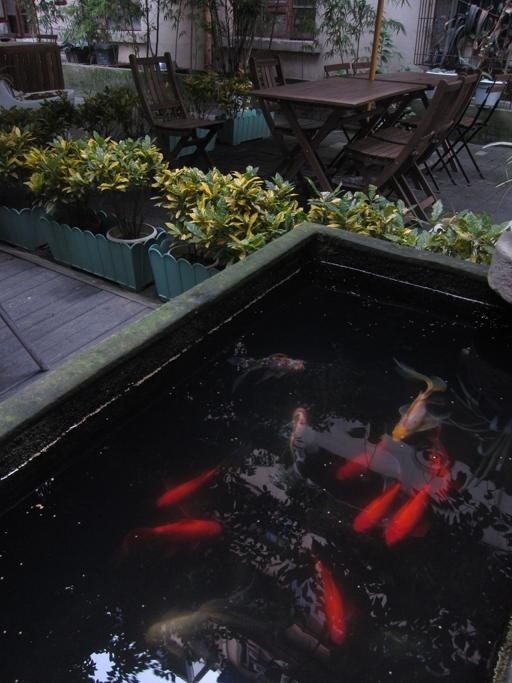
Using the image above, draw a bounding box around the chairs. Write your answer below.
[248,53,512,229]
[128,51,226,174]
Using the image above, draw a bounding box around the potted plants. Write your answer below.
[56,0,145,66]
[1,121,308,303]
[164,67,275,160]
